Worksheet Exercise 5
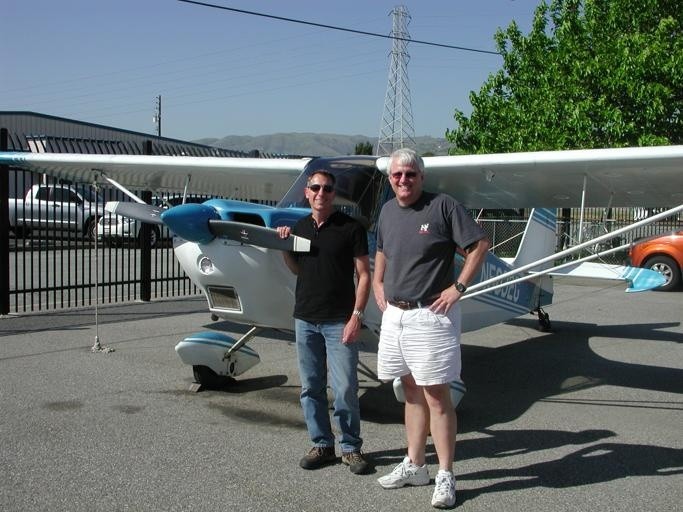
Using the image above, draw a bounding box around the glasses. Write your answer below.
[391,172,417,178]
[308,185,333,192]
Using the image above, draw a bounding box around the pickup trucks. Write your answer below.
[7,180,107,241]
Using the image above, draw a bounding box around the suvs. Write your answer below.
[98,196,207,246]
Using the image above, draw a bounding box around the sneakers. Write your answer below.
[378,456,430,488]
[431,470,456,509]
[341,450,369,474]
[300,446,335,469]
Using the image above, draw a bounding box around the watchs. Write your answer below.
[453,280,465,293]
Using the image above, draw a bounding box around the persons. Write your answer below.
[370,148,492,510]
[274,170,374,476]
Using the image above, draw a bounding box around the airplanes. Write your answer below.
[0,144,683,419]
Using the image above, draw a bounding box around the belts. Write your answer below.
[388,298,437,311]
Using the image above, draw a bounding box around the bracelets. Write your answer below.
[351,310,365,322]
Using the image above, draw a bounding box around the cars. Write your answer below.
[626,222,683,292]
